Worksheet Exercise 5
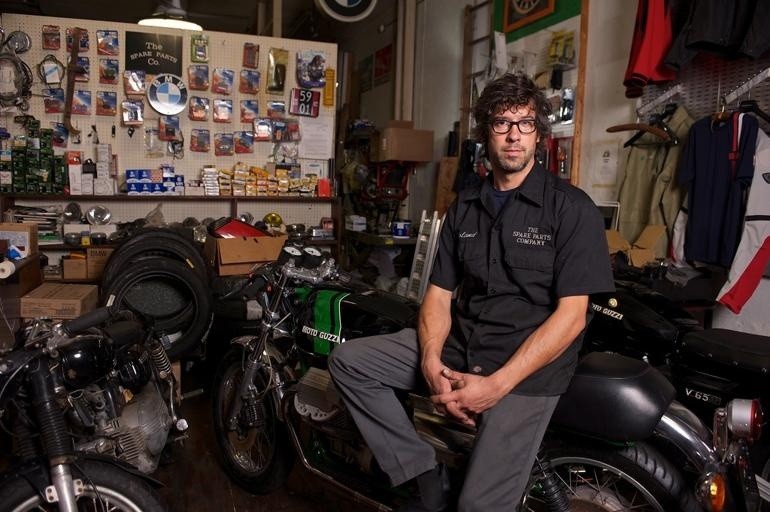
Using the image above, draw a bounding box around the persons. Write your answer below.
[325,71,618,512]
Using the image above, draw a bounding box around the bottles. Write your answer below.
[559,89,573,121]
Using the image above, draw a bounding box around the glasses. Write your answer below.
[484,117,539,134]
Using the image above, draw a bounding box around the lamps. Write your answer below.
[138,0,204,32]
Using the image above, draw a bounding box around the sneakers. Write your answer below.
[398,459,449,512]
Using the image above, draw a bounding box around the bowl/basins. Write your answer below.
[392,222,411,236]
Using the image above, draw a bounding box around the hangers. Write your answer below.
[605,67,770,149]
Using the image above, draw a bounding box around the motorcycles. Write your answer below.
[0,306,204,512]
[212,239,763,512]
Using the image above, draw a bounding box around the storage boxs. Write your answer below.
[0,221,290,320]
[363,119,436,164]
